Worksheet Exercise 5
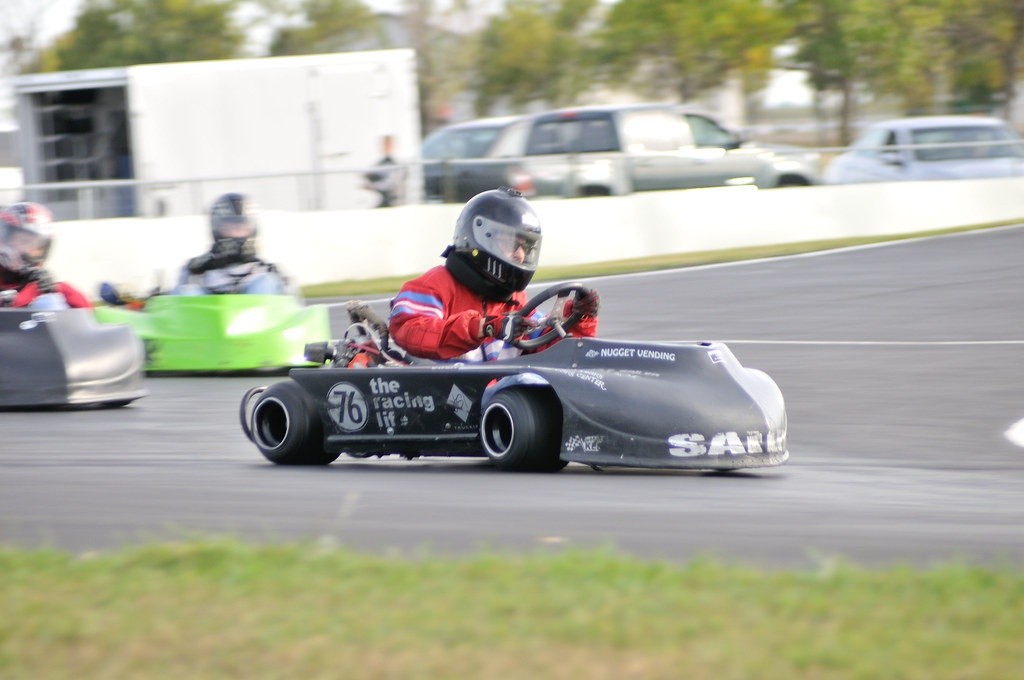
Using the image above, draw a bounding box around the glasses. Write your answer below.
[497,238,533,255]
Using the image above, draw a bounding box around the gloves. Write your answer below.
[484,314,529,342]
[573,289,600,316]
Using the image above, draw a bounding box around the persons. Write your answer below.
[0,201,96,309]
[389,187,599,366]
[186,193,289,295]
[361,134,412,209]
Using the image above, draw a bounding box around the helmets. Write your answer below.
[1,202,53,275]
[452,187,542,292]
[211,193,258,248]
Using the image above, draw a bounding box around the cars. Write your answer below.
[823,115,1024,187]
[416,107,821,207]
